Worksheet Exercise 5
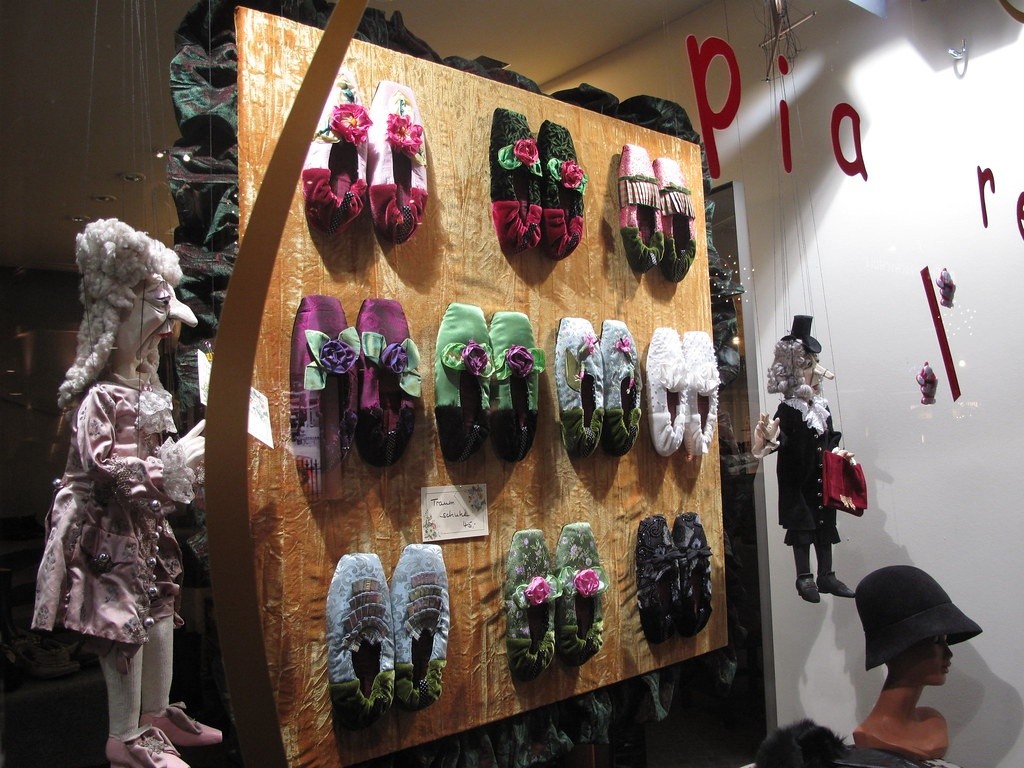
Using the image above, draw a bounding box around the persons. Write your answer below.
[751,315,858,604]
[31,221,223,768]
[856,565,982,761]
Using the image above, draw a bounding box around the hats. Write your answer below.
[855,565,983,671]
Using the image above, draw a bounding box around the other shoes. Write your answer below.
[290,296,359,473]
[505,529,563,681]
[436,302,546,467]
[555,522,603,667]
[390,544,450,707]
[370,81,428,245]
[303,67,370,230]
[672,512,713,640]
[327,552,395,723]
[618,143,696,283]
[646,328,722,458]
[557,318,643,458]
[489,108,587,260]
[635,514,682,644]
[356,298,422,466]
